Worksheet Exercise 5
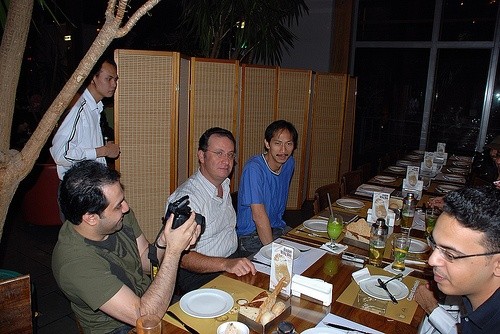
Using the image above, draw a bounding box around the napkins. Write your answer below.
[352,267,371,285]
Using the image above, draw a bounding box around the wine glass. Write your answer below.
[327,214,343,250]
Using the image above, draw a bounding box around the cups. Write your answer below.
[391,237,410,272]
[135,314,161,334]
[425,209,440,235]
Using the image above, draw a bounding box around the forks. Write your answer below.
[374,273,403,289]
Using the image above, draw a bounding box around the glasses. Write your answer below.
[200,148,237,161]
[427,235,500,263]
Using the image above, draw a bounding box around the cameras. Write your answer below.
[164,195,206,235]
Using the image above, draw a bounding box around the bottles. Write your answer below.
[401,193,415,228]
[270,322,299,334]
[369,218,389,267]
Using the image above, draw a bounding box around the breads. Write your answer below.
[261,302,285,326]
[238,305,259,321]
[225,323,242,334]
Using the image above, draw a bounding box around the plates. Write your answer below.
[390,238,430,254]
[303,219,329,232]
[374,154,420,183]
[359,275,409,300]
[179,288,234,319]
[435,157,470,194]
[260,245,301,263]
[217,321,250,334]
[336,198,364,209]
[300,327,345,334]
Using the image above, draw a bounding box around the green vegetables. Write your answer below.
[427,217,436,227]
[394,246,409,262]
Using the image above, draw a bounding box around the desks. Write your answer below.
[128,153,473,334]
[21,164,63,226]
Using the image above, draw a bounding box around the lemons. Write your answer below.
[427,227,432,233]
[370,248,379,259]
[394,264,405,267]
[395,248,407,253]
[332,220,339,225]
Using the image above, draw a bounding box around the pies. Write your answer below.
[255,277,285,321]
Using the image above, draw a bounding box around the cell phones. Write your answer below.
[341,253,366,263]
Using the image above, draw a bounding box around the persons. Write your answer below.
[13,89,48,143]
[51,161,202,334]
[236,120,298,252]
[163,127,257,293]
[415,136,500,334]
[49,58,121,225]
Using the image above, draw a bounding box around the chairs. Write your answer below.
[314,170,361,215]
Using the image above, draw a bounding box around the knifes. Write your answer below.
[377,278,398,305]
[167,310,200,334]
[323,322,372,334]
